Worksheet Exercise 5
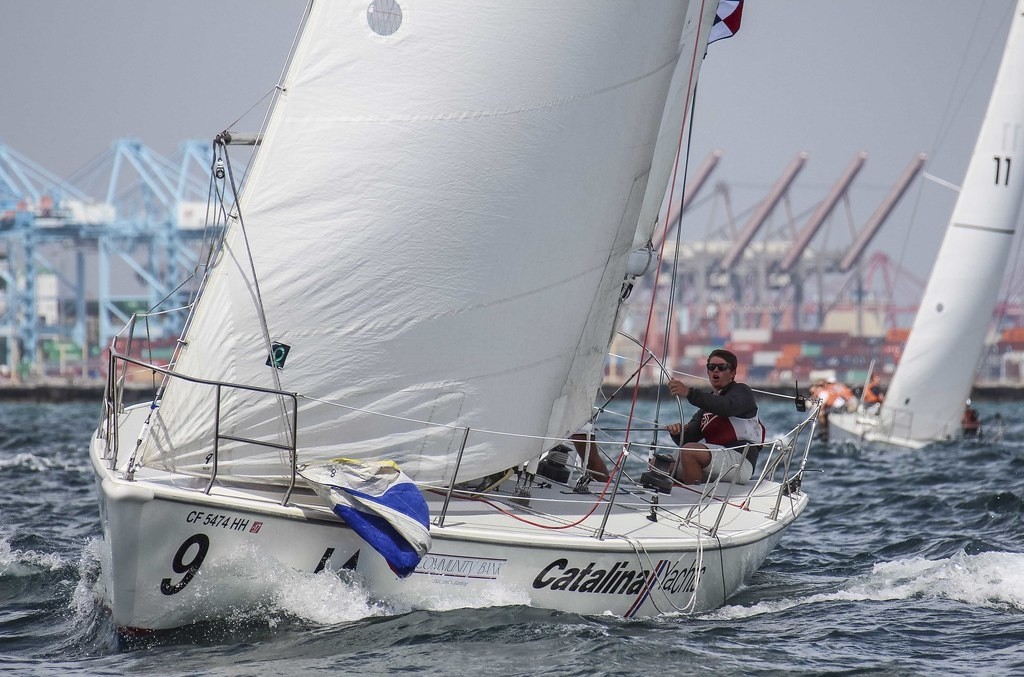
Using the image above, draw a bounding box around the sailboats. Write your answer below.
[809,0,1024,454]
[89,0,822,649]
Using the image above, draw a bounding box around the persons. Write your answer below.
[666,349,765,487]
[864,372,884,408]
[568,423,611,484]
[809,375,859,443]
[961,397,979,434]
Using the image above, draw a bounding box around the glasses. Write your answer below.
[706,363,733,371]
